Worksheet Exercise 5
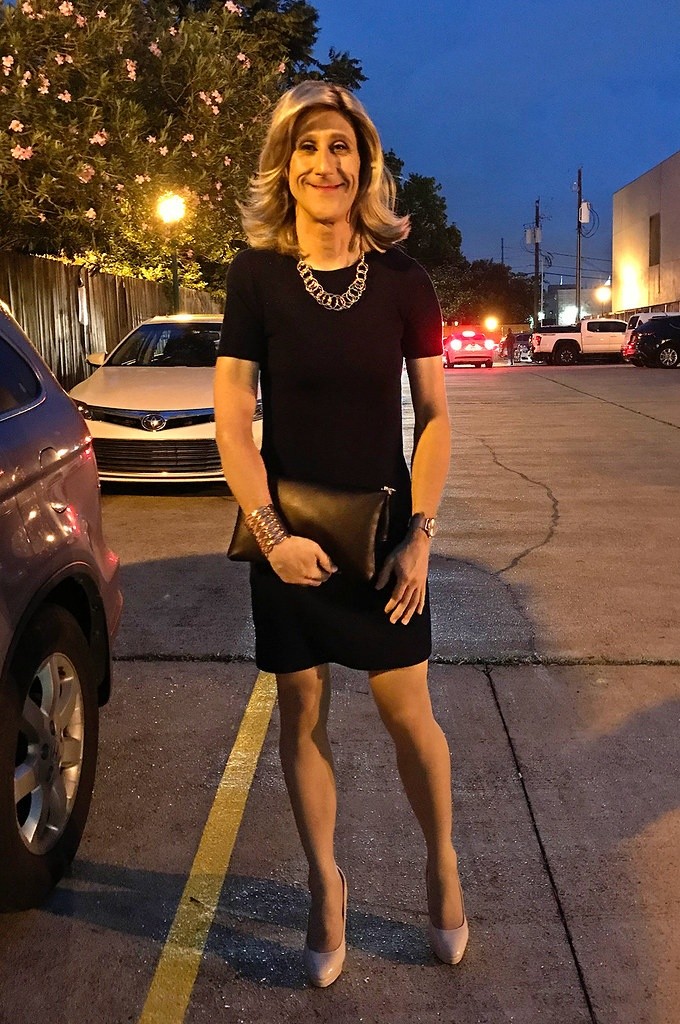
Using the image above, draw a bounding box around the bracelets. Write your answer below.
[244,503,291,560]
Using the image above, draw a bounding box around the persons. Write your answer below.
[505,328,515,365]
[214,81,469,987]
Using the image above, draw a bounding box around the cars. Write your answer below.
[69,314,264,483]
[627,314,680,369]
[619,312,680,367]
[0,296,124,900]
[442,330,494,368]
[498,332,532,358]
[520,347,544,363]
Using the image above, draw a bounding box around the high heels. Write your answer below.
[425,861,469,965]
[303,865,347,987]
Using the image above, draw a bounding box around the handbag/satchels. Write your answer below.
[226,465,397,583]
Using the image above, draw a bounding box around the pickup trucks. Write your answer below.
[530,319,628,366]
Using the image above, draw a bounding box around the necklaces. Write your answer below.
[296,242,370,311]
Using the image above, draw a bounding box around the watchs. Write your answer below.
[408,517,438,538]
